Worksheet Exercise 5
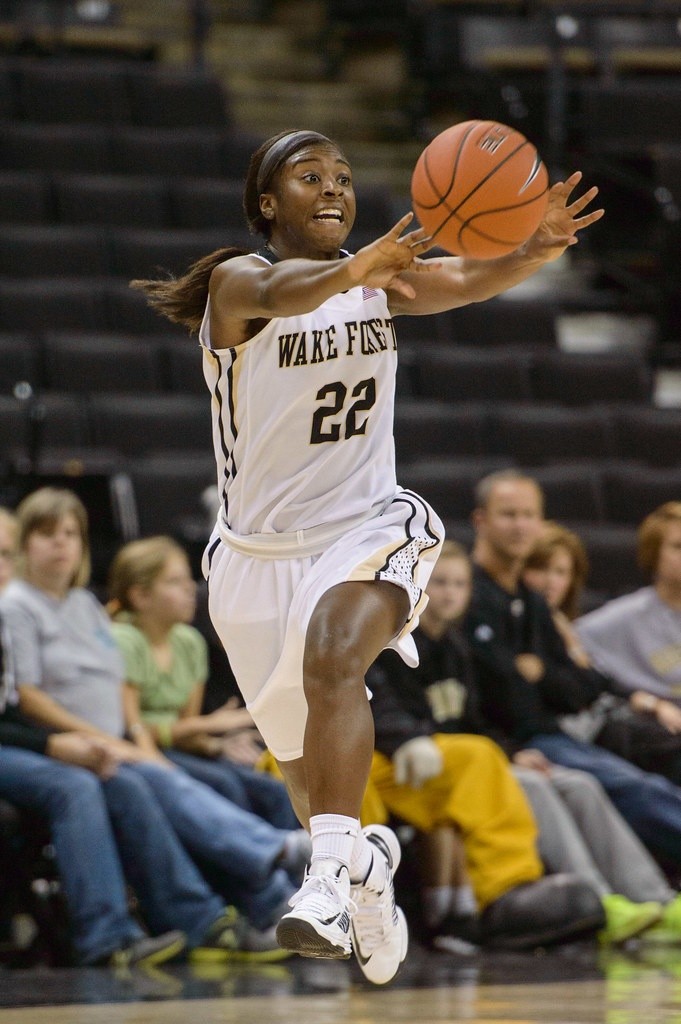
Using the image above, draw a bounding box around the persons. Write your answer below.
[131,129,605,985]
[1,468,681,966]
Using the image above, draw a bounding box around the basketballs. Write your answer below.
[408,117,552,262]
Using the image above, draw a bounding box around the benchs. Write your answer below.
[0,0,681,638]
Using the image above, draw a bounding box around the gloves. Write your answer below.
[393,737,441,784]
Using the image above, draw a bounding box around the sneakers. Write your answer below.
[276,859,359,960]
[189,906,288,962]
[347,823,403,986]
[597,894,664,946]
[108,932,187,970]
[639,895,681,943]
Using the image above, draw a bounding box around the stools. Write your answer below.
[0,799,73,966]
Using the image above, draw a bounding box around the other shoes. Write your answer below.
[433,914,484,956]
